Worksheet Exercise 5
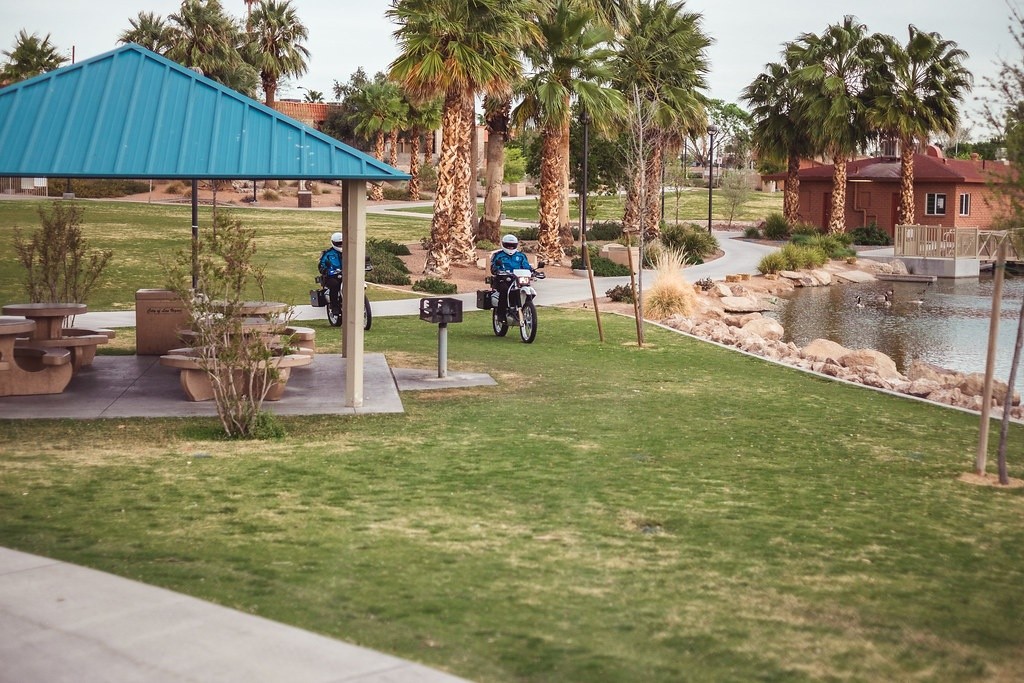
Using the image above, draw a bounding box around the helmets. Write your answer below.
[501,234,518,255]
[330,233,343,253]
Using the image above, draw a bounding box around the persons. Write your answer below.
[317,232,342,315]
[490,234,545,322]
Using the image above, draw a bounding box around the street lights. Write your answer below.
[579,104,592,269]
[705,125,717,232]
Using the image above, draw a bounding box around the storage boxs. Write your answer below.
[477,291,491,309]
[311,290,325,307]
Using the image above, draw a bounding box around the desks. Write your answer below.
[195,301,288,359]
[0,302,87,396]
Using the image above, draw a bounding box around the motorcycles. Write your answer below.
[309,255,373,329]
[476,261,546,343]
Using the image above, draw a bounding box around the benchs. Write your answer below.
[13,328,116,395]
[160,327,316,402]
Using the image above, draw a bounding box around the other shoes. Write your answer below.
[497,312,507,323]
[330,303,341,316]
